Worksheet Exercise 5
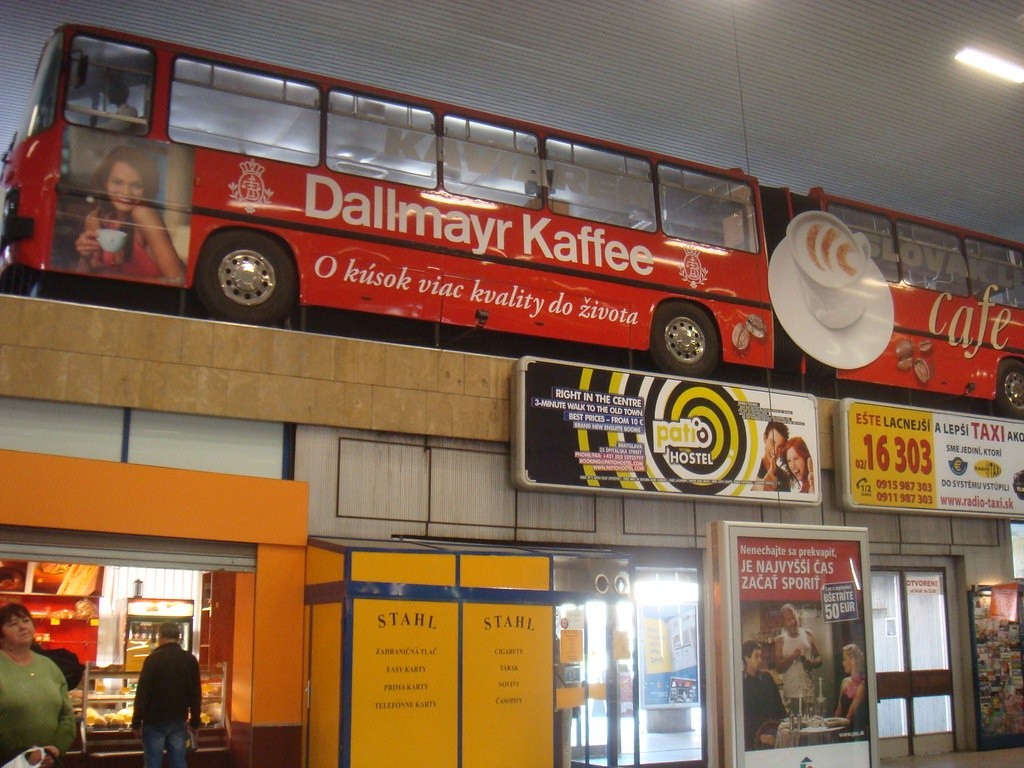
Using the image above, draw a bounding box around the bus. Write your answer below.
[768,179,1022,422]
[0,23,777,379]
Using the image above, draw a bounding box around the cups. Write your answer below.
[95,229,127,253]
[786,210,872,330]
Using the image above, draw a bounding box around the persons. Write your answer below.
[824,641,869,743]
[750,420,792,494]
[73,140,191,291]
[128,618,204,767]
[89,78,138,137]
[780,435,813,494]
[740,639,789,755]
[0,601,77,768]
[773,601,824,721]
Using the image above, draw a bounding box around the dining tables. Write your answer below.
[772,716,853,750]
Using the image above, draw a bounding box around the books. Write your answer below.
[969,595,1023,737]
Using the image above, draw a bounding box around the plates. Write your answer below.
[768,236,895,370]
